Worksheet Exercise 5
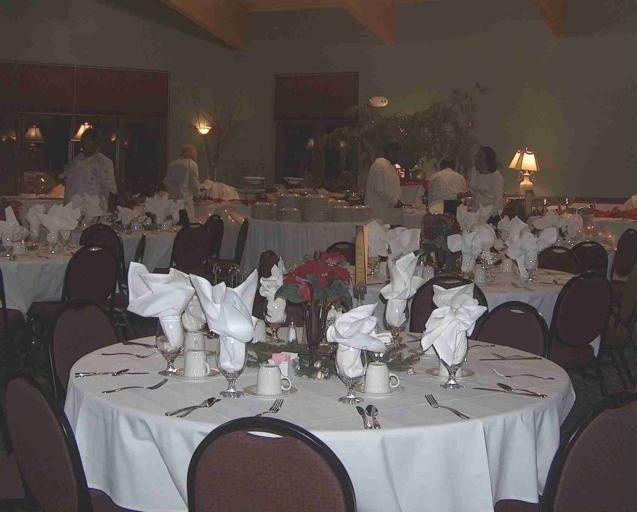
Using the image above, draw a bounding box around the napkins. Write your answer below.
[419,281,487,366]
[126,260,196,348]
[181,294,207,332]
[257,257,287,323]
[189,268,258,372]
[379,252,432,329]
[325,302,392,377]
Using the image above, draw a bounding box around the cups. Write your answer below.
[255,365,292,394]
[184,330,204,351]
[178,346,214,357]
[183,348,211,377]
[365,361,401,393]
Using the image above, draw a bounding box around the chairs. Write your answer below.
[61,214,225,317]
[476,301,549,359]
[187,417,356,512]
[548,273,612,399]
[409,226,637,338]
[2,299,120,512]
[603,268,637,391]
[494,398,637,512]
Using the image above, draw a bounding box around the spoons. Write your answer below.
[165,396,215,415]
[495,382,542,398]
[366,405,382,428]
[77,366,130,377]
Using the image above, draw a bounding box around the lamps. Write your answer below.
[507,145,540,200]
[195,126,212,137]
[23,124,45,152]
[68,122,96,152]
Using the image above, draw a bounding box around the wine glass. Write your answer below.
[261,298,288,346]
[154,315,182,377]
[460,254,539,283]
[334,347,367,404]
[214,336,249,400]
[383,299,409,352]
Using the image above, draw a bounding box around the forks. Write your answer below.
[101,350,158,358]
[102,377,168,393]
[489,367,558,381]
[253,400,283,417]
[423,394,472,420]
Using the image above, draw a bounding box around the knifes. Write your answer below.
[474,387,544,398]
[355,406,372,430]
[179,398,223,415]
[76,368,150,376]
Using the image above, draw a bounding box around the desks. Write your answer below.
[239,219,384,267]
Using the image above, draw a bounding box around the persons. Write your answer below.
[62,124,118,221]
[424,158,469,211]
[466,145,507,217]
[164,143,201,212]
[360,138,404,208]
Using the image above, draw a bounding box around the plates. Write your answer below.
[349,381,405,399]
[432,328,469,391]
[174,368,219,379]
[245,384,298,398]
[251,194,371,223]
[425,366,475,381]
